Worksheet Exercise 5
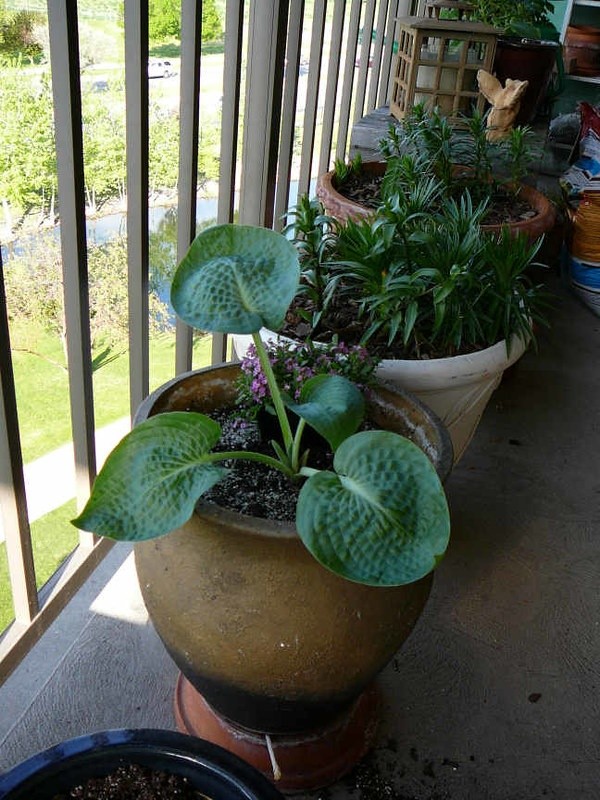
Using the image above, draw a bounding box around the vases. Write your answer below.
[132,356,455,796]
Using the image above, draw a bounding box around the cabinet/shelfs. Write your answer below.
[550,0,600,151]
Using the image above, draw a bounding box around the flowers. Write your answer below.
[69,223,450,590]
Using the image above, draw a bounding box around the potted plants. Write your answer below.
[0,729,286,800]
[222,0,563,478]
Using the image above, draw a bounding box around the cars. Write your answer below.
[351,55,375,68]
[90,81,109,93]
[146,61,179,79]
